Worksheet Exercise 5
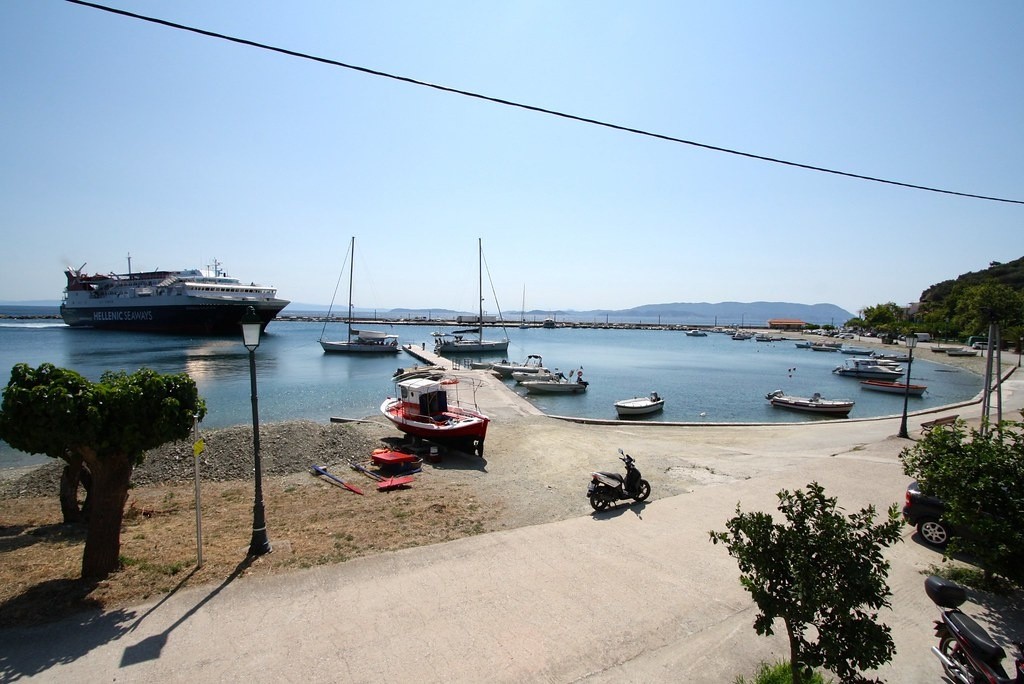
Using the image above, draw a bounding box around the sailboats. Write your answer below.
[431,237,510,352]
[316,236,404,355]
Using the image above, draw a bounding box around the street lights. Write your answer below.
[235,304,274,555]
[897,328,919,438]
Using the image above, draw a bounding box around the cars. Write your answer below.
[968,336,997,350]
[902,478,966,546]
[802,326,883,340]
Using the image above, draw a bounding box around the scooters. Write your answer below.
[586,448,651,512]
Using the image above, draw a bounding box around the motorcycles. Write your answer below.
[924,572,1023,684]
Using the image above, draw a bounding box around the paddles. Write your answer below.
[346,459,388,482]
[312,464,365,495]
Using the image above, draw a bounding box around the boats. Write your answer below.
[930,346,964,352]
[380,374,490,448]
[543,318,556,328]
[765,389,855,415]
[509,369,560,383]
[795,340,914,380]
[469,354,542,378]
[519,376,590,394]
[686,330,707,336]
[860,380,928,397]
[946,351,978,357]
[725,329,772,342]
[613,390,665,416]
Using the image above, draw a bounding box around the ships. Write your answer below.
[59,256,292,332]
